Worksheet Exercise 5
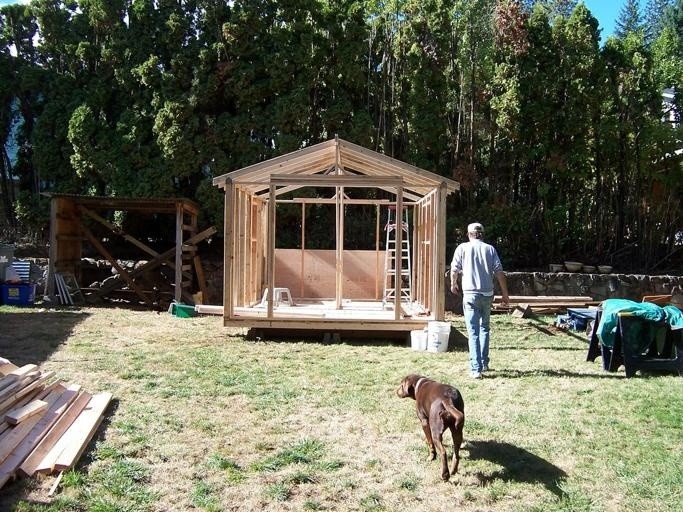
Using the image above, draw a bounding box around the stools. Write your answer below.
[259,287,295,307]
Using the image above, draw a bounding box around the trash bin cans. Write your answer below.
[1,283,35,308]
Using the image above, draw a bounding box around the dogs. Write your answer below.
[395,372,465,483]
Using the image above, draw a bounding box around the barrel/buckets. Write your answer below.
[427,321,451,353]
[410,330,427,351]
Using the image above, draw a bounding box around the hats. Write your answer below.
[468,222,485,233]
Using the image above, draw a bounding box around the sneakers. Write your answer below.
[468,366,488,378]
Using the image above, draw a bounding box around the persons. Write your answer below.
[450,221,510,378]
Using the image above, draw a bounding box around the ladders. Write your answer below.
[383,206,412,304]
